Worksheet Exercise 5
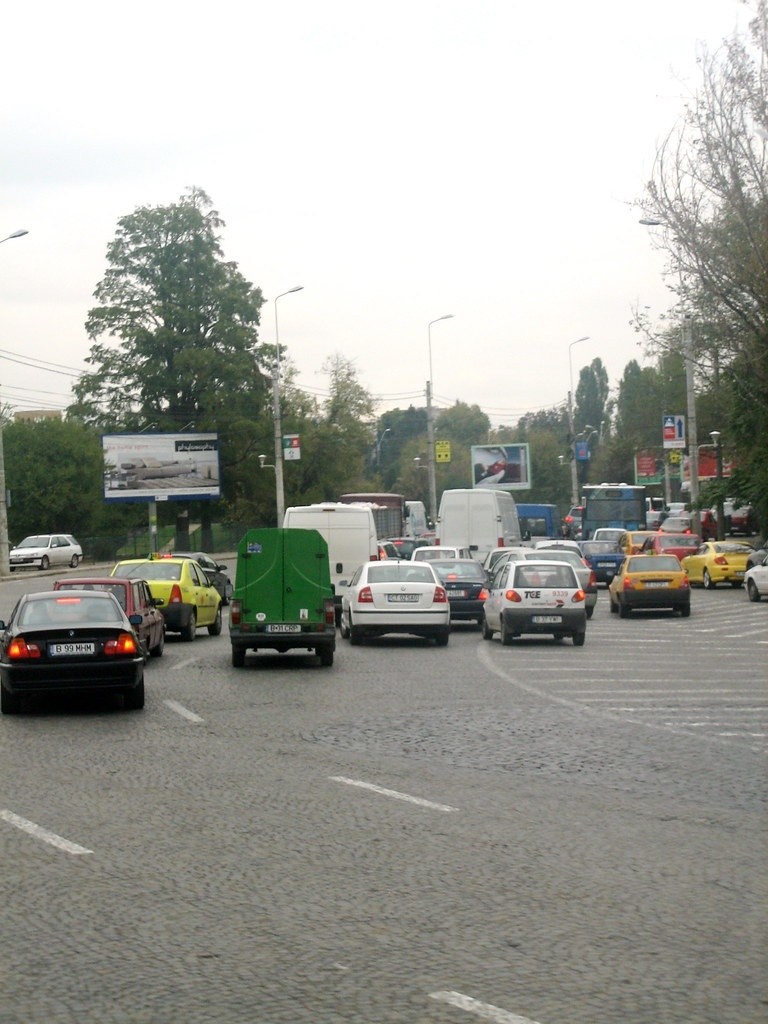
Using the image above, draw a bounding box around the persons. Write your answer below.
[562,523,568,539]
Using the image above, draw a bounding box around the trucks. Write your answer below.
[225,527,336,668]
[517,504,563,541]
[436,488,531,566]
[279,492,429,626]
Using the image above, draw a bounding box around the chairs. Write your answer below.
[87,604,107,622]
[407,574,424,582]
[545,574,559,587]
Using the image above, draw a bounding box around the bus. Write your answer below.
[581,481,647,540]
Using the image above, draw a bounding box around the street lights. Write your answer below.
[269,285,305,528]
[639,219,704,543]
[567,337,590,506]
[426,313,453,524]
[376,429,390,466]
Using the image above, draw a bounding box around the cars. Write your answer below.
[562,506,583,542]
[103,551,233,642]
[608,554,692,618]
[484,539,597,645]
[9,534,83,572]
[0,590,145,715]
[578,498,768,602]
[50,577,165,658]
[340,560,450,646]
[377,536,490,624]
